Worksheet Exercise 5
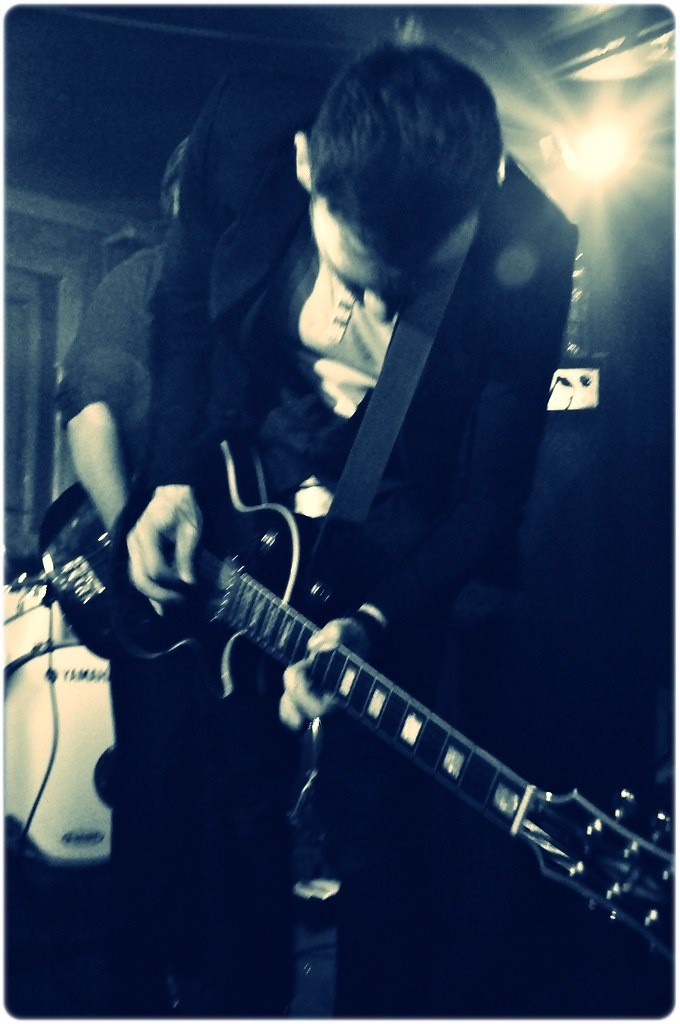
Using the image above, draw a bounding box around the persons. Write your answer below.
[56,138,189,530]
[108,42,579,1020]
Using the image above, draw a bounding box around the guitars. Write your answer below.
[38,431,675,965]
